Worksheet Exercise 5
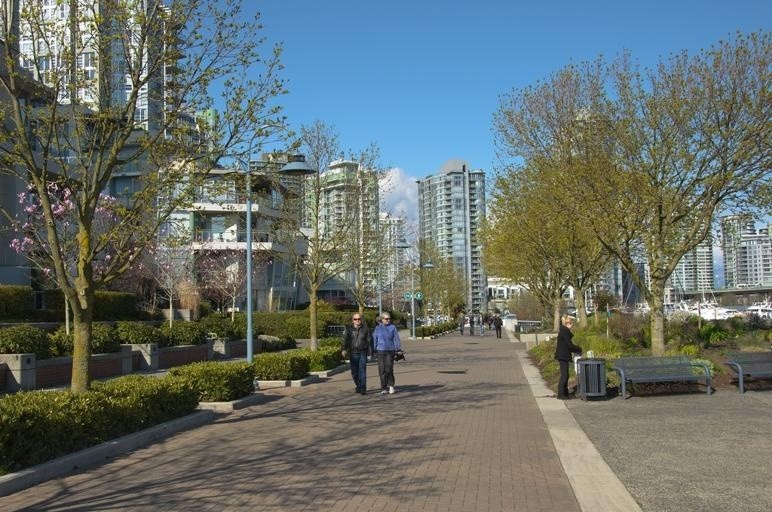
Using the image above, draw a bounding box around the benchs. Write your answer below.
[723,353,771,394]
[609,356,711,401]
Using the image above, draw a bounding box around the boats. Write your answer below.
[688,300,772,320]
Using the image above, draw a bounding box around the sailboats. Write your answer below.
[612,252,719,314]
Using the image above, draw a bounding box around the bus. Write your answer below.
[736,283,759,289]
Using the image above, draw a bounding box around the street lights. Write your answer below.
[244,124,316,390]
[411,250,435,341]
[377,221,412,315]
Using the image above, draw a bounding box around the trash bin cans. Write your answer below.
[577,358,607,401]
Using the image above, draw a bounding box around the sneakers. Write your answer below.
[557,394,569,399]
[380,385,395,394]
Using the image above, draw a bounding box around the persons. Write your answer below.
[554,314,582,400]
[341,312,403,395]
[458,312,503,338]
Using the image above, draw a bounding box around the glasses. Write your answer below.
[354,318,360,320]
[384,317,390,320]
